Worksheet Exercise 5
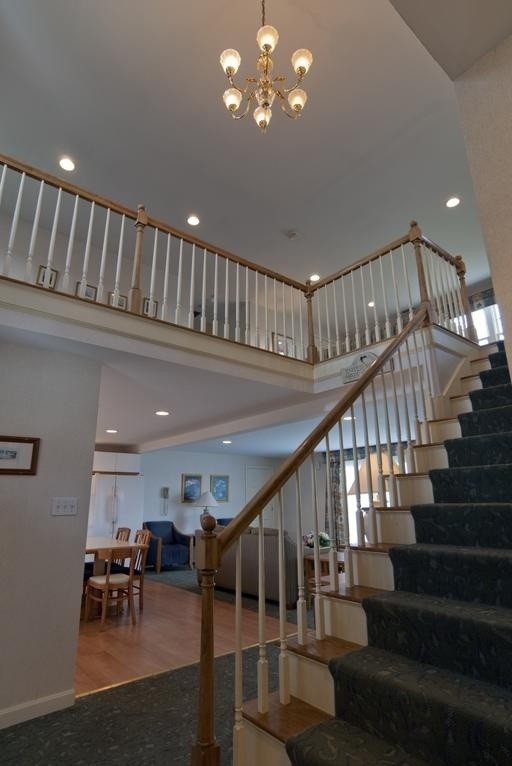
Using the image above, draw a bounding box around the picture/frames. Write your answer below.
[181,472,202,503]
[36,265,158,318]
[210,475,229,503]
[272,332,297,358]
[245,464,274,519]
[0,436,41,475]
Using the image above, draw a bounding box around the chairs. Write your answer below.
[143,521,194,575]
[80,528,152,625]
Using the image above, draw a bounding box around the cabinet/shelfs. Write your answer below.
[85,451,144,572]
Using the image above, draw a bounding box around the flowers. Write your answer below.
[302,528,331,548]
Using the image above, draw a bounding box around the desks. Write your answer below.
[303,551,347,607]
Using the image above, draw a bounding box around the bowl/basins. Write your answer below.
[301,545,331,554]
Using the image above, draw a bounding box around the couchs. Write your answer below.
[210,516,330,610]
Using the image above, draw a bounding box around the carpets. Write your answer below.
[1,630,296,766]
[144,565,315,630]
[285,341,512,766]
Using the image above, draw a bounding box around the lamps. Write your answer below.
[220,0,314,136]
[347,446,404,508]
[194,491,219,520]
